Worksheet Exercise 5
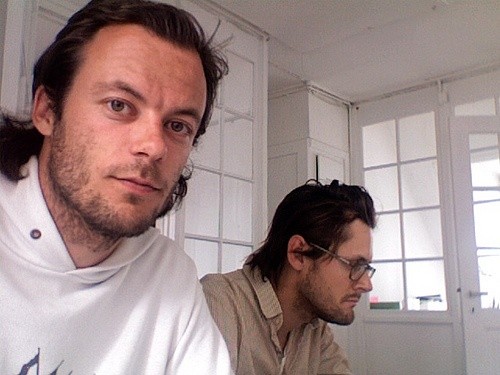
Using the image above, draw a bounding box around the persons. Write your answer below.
[0,0,238,375]
[199,179,377,375]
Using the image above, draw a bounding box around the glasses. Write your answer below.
[309,242,376,281]
